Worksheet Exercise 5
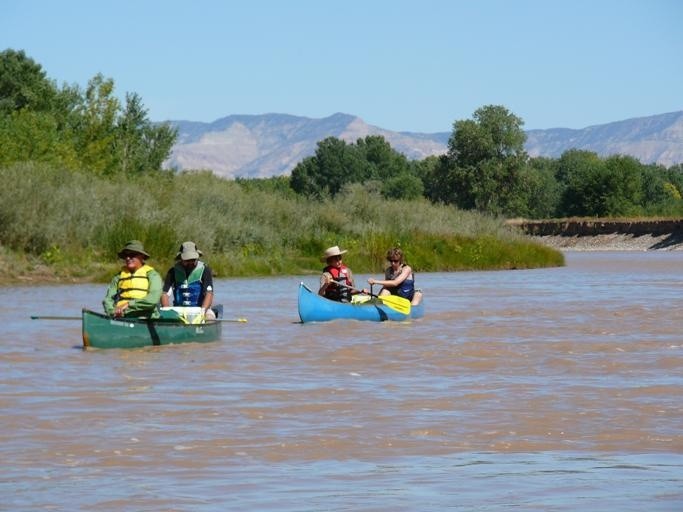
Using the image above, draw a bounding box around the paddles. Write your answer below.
[329,278,411,316]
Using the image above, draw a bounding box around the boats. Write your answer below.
[79,302,224,352]
[295,280,424,325]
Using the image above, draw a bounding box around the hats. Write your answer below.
[176,241,202,260]
[320,246,348,263]
[118,240,149,259]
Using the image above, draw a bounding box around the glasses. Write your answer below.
[390,260,399,262]
[125,253,138,259]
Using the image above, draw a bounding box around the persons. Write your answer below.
[367,247,415,306]
[159,241,216,325]
[102,240,163,321]
[317,245,370,304]
